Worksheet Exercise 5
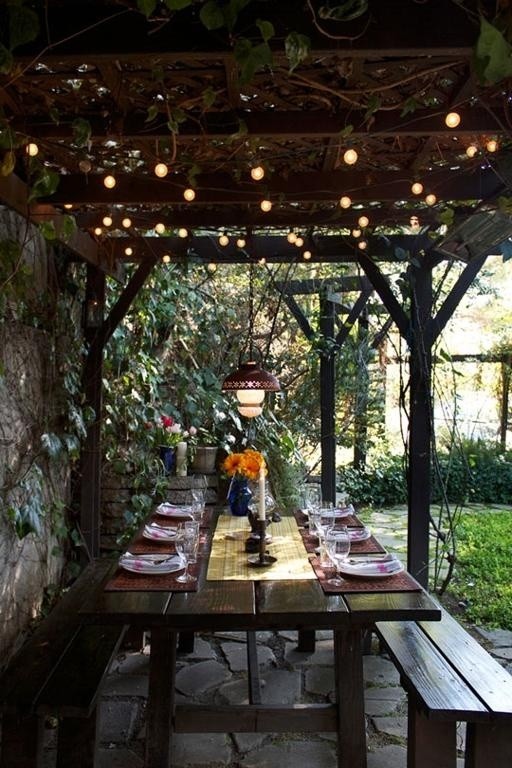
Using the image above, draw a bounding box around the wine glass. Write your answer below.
[174,472,207,583]
[298,482,351,587]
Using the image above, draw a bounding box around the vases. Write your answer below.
[158,439,179,480]
[227,469,255,518]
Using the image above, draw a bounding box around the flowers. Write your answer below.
[223,445,264,481]
[155,414,196,443]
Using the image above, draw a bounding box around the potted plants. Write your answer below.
[188,423,220,475]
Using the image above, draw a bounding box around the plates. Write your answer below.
[338,555,405,578]
[315,525,372,544]
[142,525,182,542]
[155,504,195,519]
[303,506,355,520]
[121,554,186,575]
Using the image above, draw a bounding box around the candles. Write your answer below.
[258,461,268,522]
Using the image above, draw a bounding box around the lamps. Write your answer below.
[221,186,280,418]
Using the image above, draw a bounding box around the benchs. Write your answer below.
[0,552,147,768]
[375,598,512,768]
